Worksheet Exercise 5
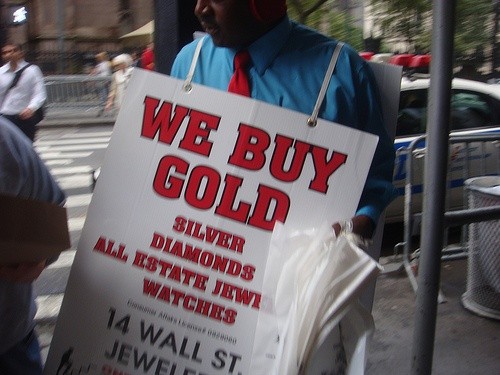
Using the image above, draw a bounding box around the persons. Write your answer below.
[0,42,47,143]
[0,0,394,375]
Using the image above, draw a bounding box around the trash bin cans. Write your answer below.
[459,175,500,320]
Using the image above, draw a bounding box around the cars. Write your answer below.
[357,51,500,227]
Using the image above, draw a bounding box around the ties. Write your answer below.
[228,50,254,97]
